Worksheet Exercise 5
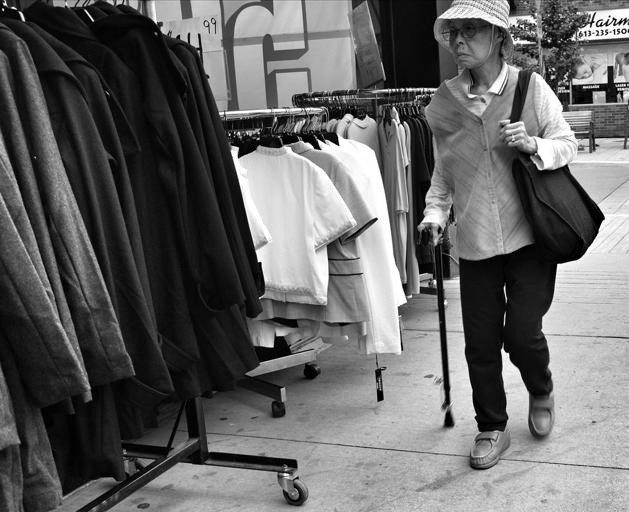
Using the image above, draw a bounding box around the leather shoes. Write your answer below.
[527,390,556,441]
[469,423,511,470]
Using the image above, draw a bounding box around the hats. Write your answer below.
[433,1,516,59]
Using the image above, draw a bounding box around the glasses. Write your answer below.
[440,24,492,43]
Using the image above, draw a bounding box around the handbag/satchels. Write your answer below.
[503,69,603,267]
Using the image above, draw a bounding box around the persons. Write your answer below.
[417,0,578,469]
[570,57,607,84]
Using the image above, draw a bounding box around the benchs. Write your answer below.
[561,109,596,152]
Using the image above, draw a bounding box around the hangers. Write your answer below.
[1,0,142,28]
[214,83,431,151]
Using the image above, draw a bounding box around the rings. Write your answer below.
[512,134,515,143]
